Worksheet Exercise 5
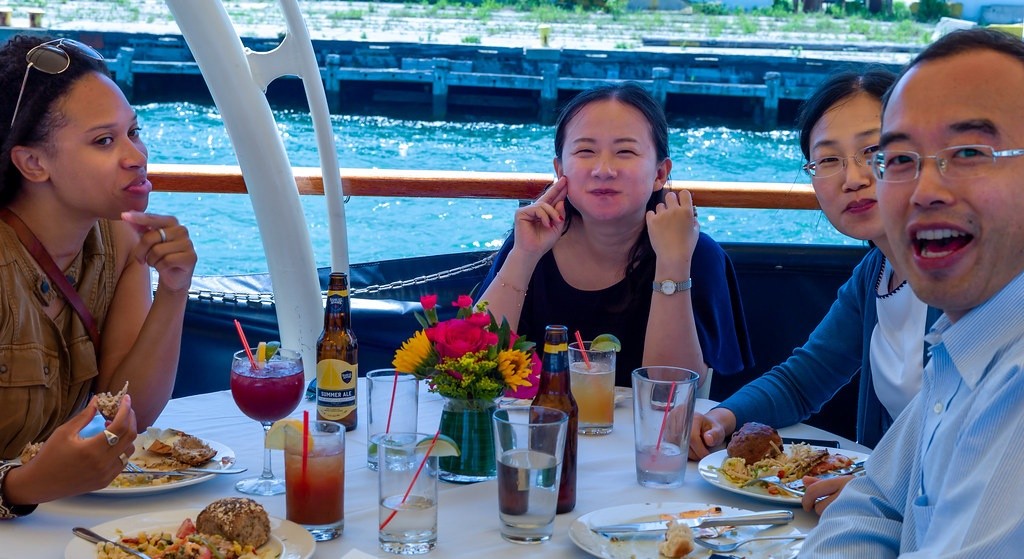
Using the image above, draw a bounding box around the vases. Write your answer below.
[428,398,511,483]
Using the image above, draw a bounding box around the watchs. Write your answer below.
[652,278,692,295]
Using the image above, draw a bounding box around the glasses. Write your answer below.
[803,143,882,179]
[867,144,1024,183]
[11,38,103,129]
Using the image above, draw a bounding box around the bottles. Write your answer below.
[527,325,578,514]
[314,273,359,433]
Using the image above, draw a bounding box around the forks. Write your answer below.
[694,535,807,553]
[125,462,214,474]
[739,475,823,500]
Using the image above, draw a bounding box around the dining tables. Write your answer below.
[0,375,872,559]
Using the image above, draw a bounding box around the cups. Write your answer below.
[284,419,346,541]
[632,366,699,490]
[366,368,419,472]
[493,407,569,545]
[567,341,616,435]
[378,433,439,554]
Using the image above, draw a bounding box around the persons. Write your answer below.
[461,81,753,399]
[0,36,197,520]
[668,69,945,518]
[796,26,1024,559]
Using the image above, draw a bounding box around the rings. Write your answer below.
[119,453,128,466]
[159,228,166,242]
[104,429,118,446]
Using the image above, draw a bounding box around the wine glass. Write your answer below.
[230,347,305,495]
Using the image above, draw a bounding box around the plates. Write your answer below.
[698,444,870,504]
[568,502,805,559]
[64,508,316,559]
[89,434,234,494]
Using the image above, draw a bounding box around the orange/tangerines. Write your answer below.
[256,342,266,369]
[264,419,314,453]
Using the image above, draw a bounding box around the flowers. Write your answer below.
[393,295,542,402]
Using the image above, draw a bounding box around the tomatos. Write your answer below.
[176,518,198,539]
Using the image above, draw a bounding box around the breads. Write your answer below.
[171,435,218,464]
[726,422,783,464]
[97,380,129,422]
[195,497,271,549]
[659,520,695,559]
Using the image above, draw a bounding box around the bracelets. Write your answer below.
[0,463,38,519]
[496,272,528,295]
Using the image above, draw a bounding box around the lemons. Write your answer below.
[414,434,461,457]
[590,334,621,352]
[265,341,280,362]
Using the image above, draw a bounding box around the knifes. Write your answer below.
[785,461,865,488]
[591,511,794,533]
[121,468,248,475]
